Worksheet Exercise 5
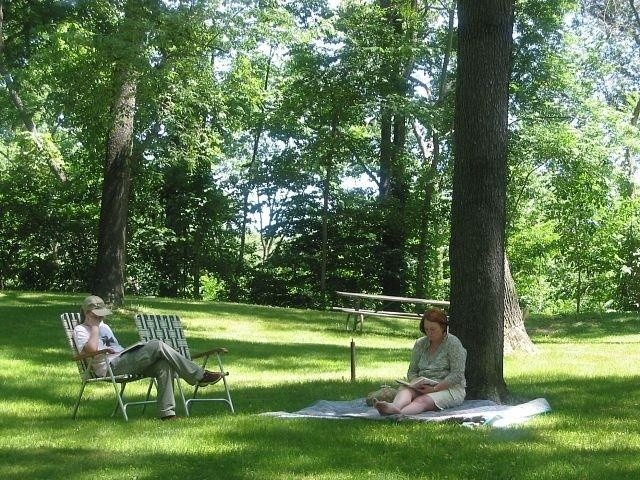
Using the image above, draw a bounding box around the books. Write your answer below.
[394,376,440,390]
[117,341,149,356]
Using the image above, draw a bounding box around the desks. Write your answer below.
[335,287,451,333]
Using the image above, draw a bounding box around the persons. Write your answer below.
[372,309,468,417]
[72,295,221,422]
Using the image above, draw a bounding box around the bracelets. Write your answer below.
[434,385,437,392]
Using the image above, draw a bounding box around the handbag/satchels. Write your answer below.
[366,388,398,406]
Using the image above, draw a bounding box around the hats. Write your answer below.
[82,294,113,317]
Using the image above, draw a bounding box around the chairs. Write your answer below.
[59,310,237,423]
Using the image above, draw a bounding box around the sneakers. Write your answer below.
[162,415,180,421]
[199,372,222,387]
